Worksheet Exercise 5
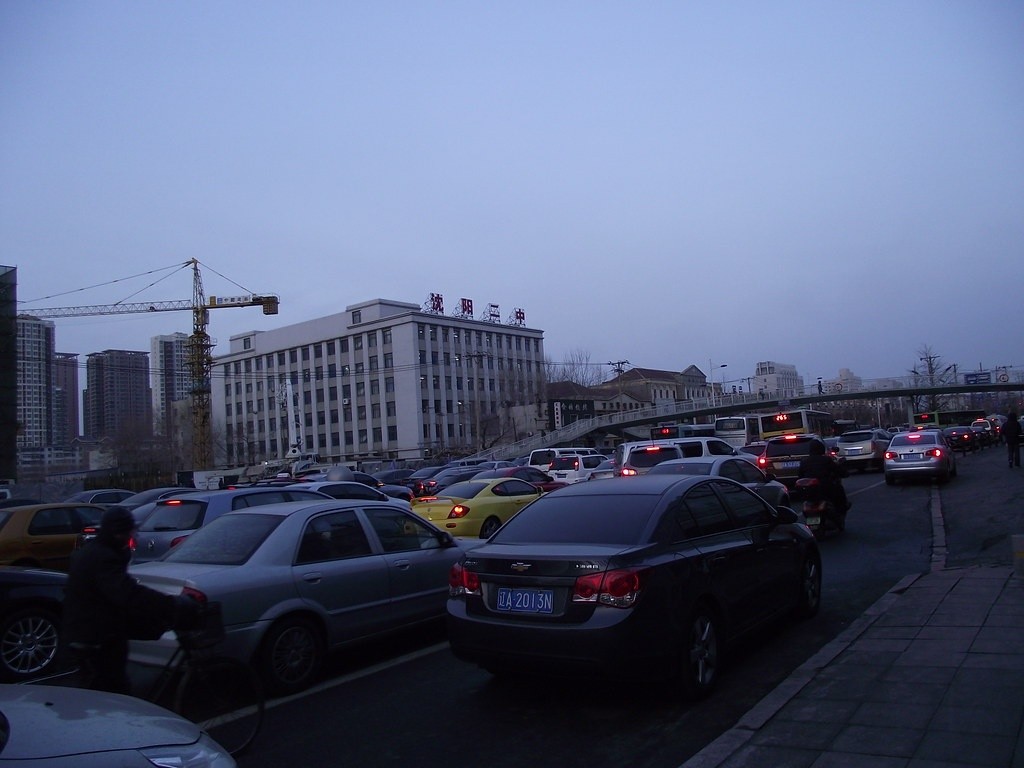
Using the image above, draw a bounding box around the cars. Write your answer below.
[0,498,47,508]
[0,504,105,568]
[69,499,487,695]
[0,565,73,681]
[117,487,203,528]
[62,489,137,504]
[446,473,822,705]
[883,429,958,485]
[123,486,337,569]
[297,416,1006,540]
[0,684,238,768]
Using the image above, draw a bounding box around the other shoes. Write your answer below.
[1009,460,1013,468]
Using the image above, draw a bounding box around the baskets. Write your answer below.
[176,601,226,650]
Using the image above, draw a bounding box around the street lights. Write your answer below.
[710,364,728,419]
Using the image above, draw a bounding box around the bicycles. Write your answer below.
[16,600,265,757]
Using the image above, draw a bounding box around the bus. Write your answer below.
[912,410,986,430]
[650,424,714,439]
[758,409,838,440]
[714,416,759,447]
[834,420,861,437]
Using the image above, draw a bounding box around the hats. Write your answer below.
[103,506,135,531]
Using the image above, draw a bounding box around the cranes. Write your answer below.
[16,258,281,471]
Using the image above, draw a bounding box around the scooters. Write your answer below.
[796,457,852,544]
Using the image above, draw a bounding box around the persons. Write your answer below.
[540,429,547,444]
[798,440,852,519]
[61,505,170,693]
[1001,412,1022,468]
[818,381,825,394]
